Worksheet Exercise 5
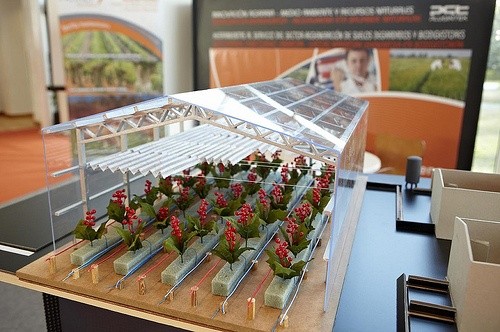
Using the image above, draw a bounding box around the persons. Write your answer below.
[340,49,378,94]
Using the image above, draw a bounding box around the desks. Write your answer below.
[0,172,458,332]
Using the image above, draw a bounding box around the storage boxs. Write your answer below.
[447,216,500,332]
[431,168,500,241]
